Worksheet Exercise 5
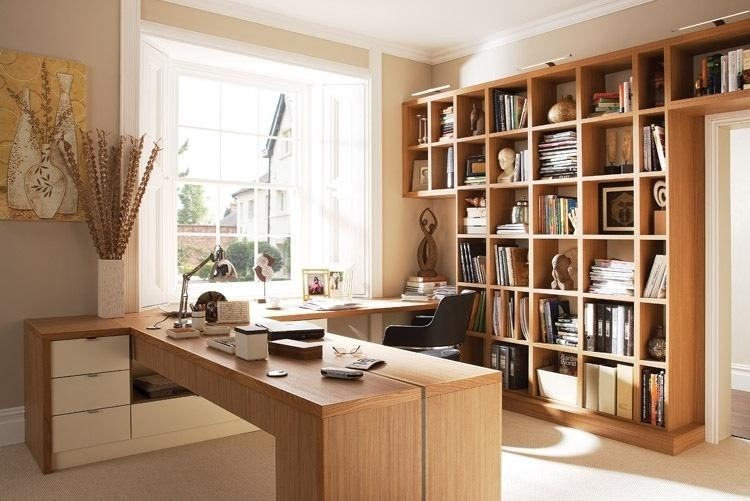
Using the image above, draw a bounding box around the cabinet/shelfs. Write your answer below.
[403,20,749,458]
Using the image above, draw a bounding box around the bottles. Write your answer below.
[647,324,667,361]
[511,196,529,225]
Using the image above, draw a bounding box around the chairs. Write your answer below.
[380,293,477,350]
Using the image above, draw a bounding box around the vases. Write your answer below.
[98,259,123,319]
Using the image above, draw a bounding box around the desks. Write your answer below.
[24,293,504,499]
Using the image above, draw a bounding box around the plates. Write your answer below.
[266,307,282,310]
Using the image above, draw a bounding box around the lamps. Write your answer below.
[173,245,238,328]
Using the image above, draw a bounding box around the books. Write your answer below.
[468,291,529,340]
[514,150,528,182]
[642,368,664,426]
[642,124,665,171]
[538,195,577,235]
[460,242,486,283]
[494,244,529,287]
[586,77,633,118]
[648,70,664,108]
[493,88,528,132]
[694,48,750,96]
[538,298,579,347]
[402,276,456,302]
[589,255,666,298]
[463,208,528,235]
[438,105,453,141]
[538,130,577,180]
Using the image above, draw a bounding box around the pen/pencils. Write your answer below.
[190,303,207,312]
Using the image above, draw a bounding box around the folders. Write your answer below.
[584,303,634,356]
[491,343,528,390]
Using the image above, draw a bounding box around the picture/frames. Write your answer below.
[303,269,347,301]
[598,181,634,235]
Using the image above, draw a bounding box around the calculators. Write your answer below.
[345,357,385,370]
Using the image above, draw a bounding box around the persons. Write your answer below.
[422,169,428,184]
[551,254,574,291]
[497,148,515,179]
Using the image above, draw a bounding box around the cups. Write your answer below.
[464,197,487,207]
[270,297,280,308]
[192,311,205,332]
